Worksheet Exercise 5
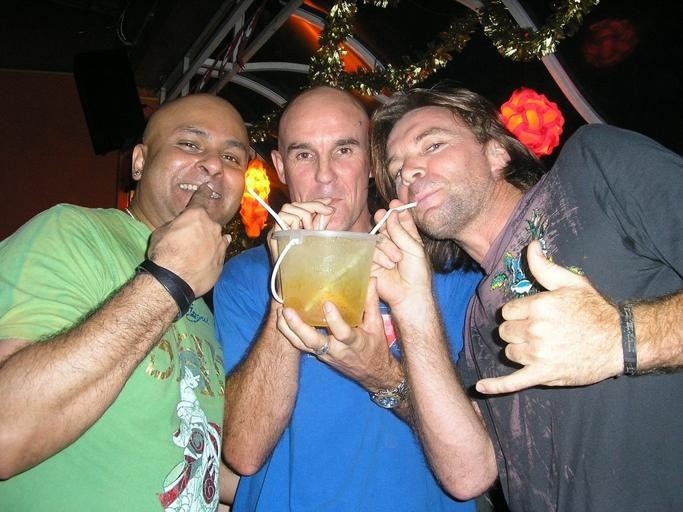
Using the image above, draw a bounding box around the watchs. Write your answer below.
[366,368,410,411]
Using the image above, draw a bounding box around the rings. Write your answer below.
[313,342,329,356]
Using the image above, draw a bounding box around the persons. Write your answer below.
[368,81,683,510]
[210,85,491,510]
[0,91,251,509]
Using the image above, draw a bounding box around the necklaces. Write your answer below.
[123,208,136,220]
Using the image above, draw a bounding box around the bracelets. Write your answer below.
[614,299,639,377]
[132,258,195,323]
